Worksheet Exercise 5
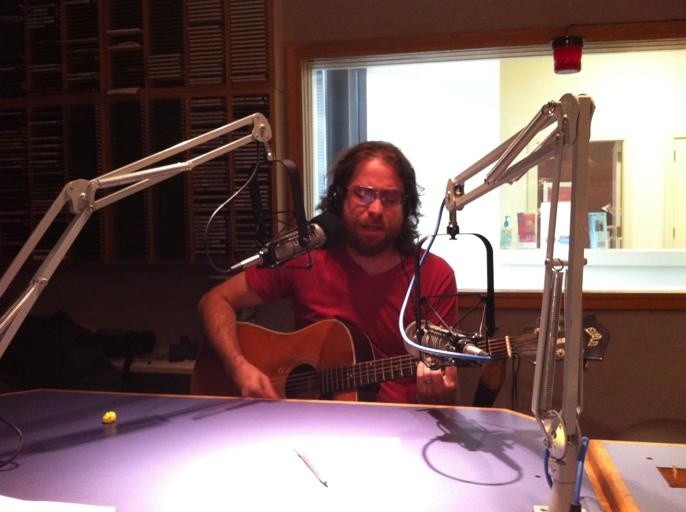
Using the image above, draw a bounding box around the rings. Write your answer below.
[423,378,433,386]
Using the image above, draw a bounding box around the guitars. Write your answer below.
[191,316,609,403]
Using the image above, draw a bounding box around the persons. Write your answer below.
[194,138,461,411]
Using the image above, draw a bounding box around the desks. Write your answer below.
[0,389,686,510]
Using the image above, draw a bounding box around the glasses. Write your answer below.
[345,186,404,207]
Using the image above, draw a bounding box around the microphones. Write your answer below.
[402,318,487,366]
[225,211,345,276]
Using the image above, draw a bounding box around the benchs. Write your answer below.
[110,351,199,375]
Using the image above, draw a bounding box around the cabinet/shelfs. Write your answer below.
[0,0,277,267]
